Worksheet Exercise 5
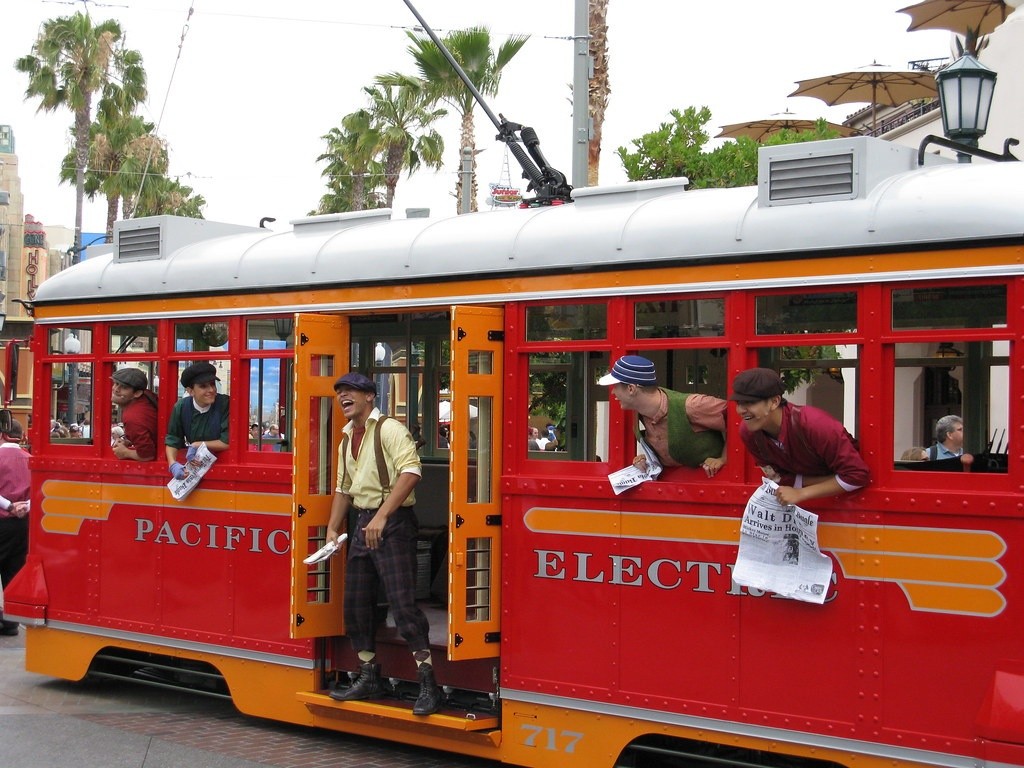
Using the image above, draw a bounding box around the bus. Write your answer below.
[0,136,1024,768]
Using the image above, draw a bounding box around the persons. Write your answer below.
[326,373,444,715]
[731,368,869,505]
[249,424,281,452]
[0,419,31,635]
[901,415,963,461]
[50,415,89,438]
[599,355,728,478]
[528,423,558,451]
[109,369,158,461]
[440,424,476,449]
[165,362,230,480]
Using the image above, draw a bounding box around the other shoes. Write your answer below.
[0,622,19,636]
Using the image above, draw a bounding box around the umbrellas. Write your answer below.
[787,60,939,138]
[895,0,1015,37]
[713,108,861,145]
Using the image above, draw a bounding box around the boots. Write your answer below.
[329,662,388,701]
[413,670,443,714]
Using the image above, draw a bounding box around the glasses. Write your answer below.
[920,456,930,462]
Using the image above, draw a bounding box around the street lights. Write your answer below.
[934,50,998,455]
[373,342,386,413]
[64,332,80,426]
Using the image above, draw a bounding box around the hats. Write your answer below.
[109,367,148,390]
[181,363,221,389]
[333,372,377,398]
[730,368,785,403]
[70,425,79,432]
[597,356,658,388]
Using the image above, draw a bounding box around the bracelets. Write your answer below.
[6,502,14,512]
